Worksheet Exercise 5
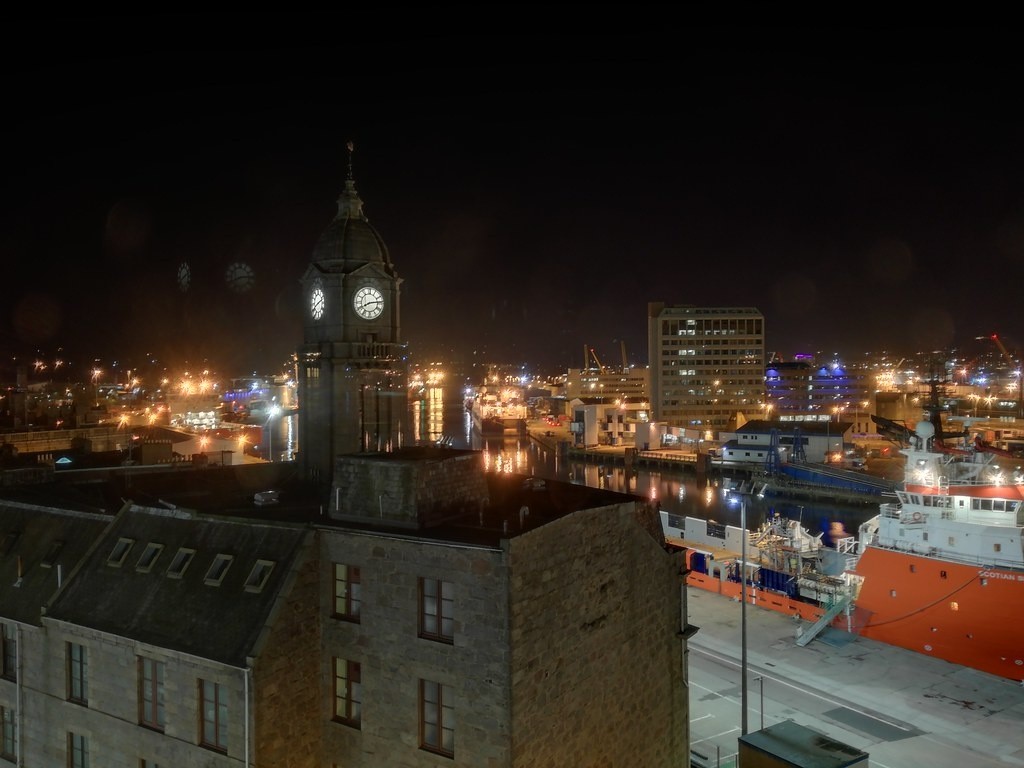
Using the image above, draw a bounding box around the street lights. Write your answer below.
[725,492,758,734]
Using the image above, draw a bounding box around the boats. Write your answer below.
[661,422,1023,684]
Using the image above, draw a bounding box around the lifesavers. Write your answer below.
[912,511,921,520]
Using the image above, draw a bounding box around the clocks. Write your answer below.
[310,288,325,319]
[354,286,384,320]
